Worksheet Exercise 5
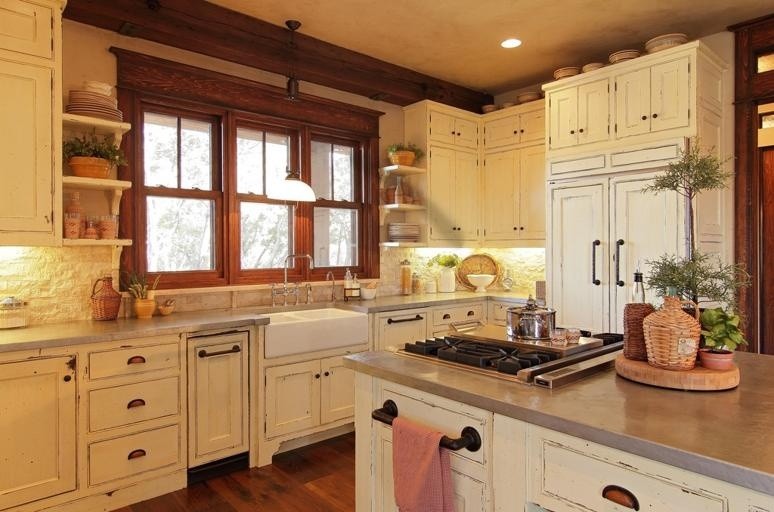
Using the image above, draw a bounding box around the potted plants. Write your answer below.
[63,133,128,179]
[427,253,463,292]
[701,309,748,368]
[388,141,423,165]
[638,136,753,349]
[127,273,164,320]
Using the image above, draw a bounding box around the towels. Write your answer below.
[392,417,455,512]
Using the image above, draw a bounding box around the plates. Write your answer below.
[66,78,123,122]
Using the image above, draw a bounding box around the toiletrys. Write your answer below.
[344,268,352,297]
[352,273,360,297]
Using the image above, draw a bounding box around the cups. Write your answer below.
[63,213,119,238]
[549,326,582,346]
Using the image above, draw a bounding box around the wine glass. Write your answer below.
[467,275,495,293]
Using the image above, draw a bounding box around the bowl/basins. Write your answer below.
[359,287,377,300]
[552,32,691,81]
[387,222,421,242]
[481,79,550,115]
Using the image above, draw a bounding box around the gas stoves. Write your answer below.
[397,321,625,390]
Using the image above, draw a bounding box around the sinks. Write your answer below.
[260,308,369,358]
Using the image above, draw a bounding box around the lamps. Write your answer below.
[268,19,315,203]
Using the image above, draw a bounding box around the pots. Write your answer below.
[507,295,558,341]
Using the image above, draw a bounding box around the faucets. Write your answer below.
[272,253,313,307]
[326,271,336,301]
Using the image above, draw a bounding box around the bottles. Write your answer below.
[343,267,360,297]
[382,175,422,204]
[400,258,424,296]
[68,191,86,238]
[0,296,30,331]
[631,271,645,303]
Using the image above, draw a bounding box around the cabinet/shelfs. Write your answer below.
[262,345,370,442]
[379,99,545,247]
[528,424,727,512]
[375,308,429,352]
[543,39,727,334]
[188,331,248,470]
[0,353,76,512]
[1,0,133,246]
[87,341,180,487]
[431,300,488,338]
[488,299,524,326]
[379,381,493,512]
